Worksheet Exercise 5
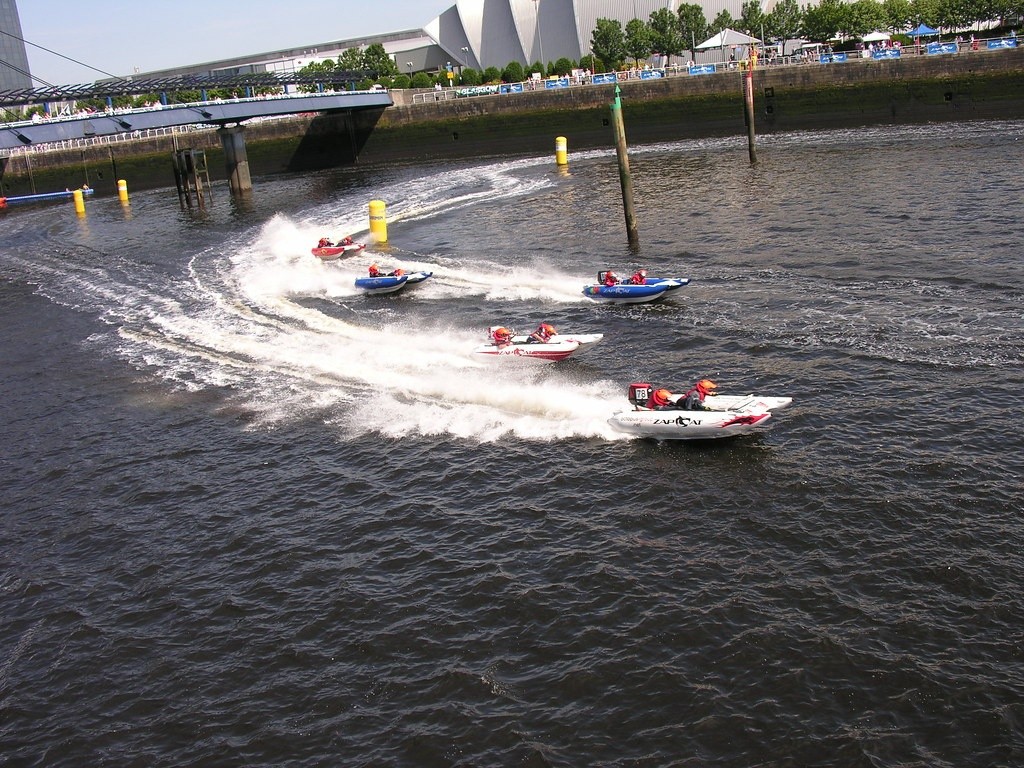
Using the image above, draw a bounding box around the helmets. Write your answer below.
[371,265,378,272]
[398,269,405,275]
[608,272,617,280]
[656,389,672,400]
[638,270,646,277]
[497,328,509,337]
[699,380,717,391]
[544,325,557,336]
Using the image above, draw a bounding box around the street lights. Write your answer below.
[461,47,469,69]
[407,61,412,79]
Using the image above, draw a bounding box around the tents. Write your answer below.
[862,31,890,51]
[692,23,766,67]
[904,23,939,46]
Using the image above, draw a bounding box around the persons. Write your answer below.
[1009,29,1016,37]
[757,48,778,68]
[868,41,899,57]
[629,62,654,78]
[369,263,380,276]
[318,238,328,248]
[827,45,833,53]
[684,59,694,66]
[676,379,715,411]
[435,82,442,91]
[297,86,346,93]
[209,91,291,100]
[671,61,677,69]
[493,328,511,349]
[803,48,815,63]
[338,237,353,246]
[527,323,551,344]
[31,100,162,125]
[646,388,673,409]
[631,269,646,285]
[604,272,624,286]
[388,268,405,276]
[527,76,540,90]
[370,85,376,91]
[560,67,591,84]
[954,33,974,50]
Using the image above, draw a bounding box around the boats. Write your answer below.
[310,244,366,262]
[351,271,433,294]
[0,189,94,209]
[582,270,692,303]
[607,383,796,440]
[474,326,605,362]
